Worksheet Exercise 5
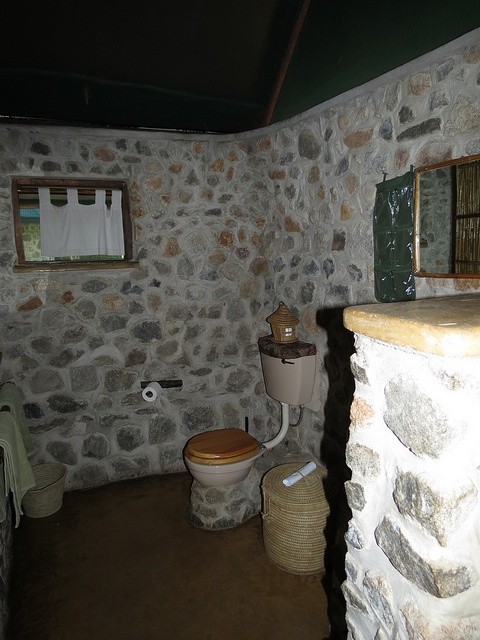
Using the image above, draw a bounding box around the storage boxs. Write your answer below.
[24,464,68,518]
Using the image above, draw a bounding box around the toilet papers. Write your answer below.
[140,382,166,403]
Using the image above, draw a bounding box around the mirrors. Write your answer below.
[414,154,480,279]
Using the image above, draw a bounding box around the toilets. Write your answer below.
[184,337,316,530]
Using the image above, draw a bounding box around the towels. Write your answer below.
[0,383,34,447]
[0,411,36,529]
